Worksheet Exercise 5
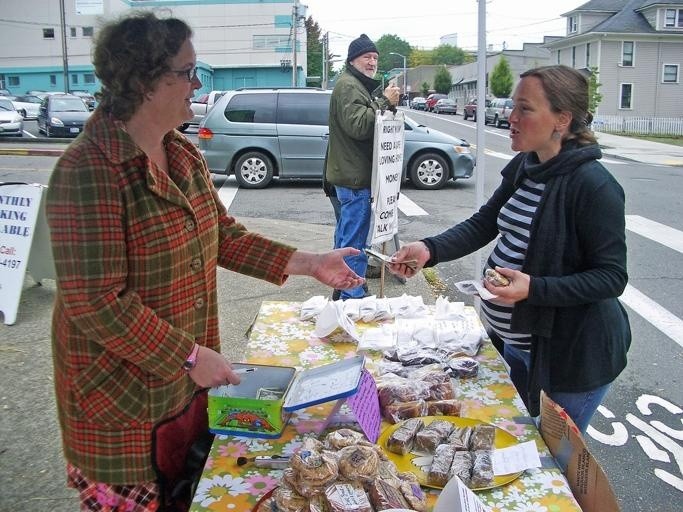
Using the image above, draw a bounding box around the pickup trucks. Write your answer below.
[177,90,227,132]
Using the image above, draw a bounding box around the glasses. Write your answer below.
[167,65,199,83]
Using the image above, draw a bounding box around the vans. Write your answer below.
[195,87,476,190]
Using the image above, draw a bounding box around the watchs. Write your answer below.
[183,344,199,373]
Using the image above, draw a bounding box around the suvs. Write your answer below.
[462,98,492,122]
[485,98,515,129]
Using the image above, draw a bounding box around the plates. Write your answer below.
[373,416,530,492]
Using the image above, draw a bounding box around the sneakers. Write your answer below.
[331,282,369,301]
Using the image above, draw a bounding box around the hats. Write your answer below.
[347,36,379,63]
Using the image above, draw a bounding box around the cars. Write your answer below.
[0,90,102,139]
[401,90,456,116]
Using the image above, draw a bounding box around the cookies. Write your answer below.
[270,364,495,511]
[484,268,510,288]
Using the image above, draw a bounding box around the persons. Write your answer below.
[43,8,368,512]
[385,65,631,433]
[322,34,400,299]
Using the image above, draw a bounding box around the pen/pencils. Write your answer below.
[234,368,259,375]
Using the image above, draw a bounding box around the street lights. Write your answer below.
[388,52,405,95]
[320,35,342,89]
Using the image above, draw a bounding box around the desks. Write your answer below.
[190,302,583,512]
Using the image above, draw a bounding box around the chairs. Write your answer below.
[152,385,210,512]
